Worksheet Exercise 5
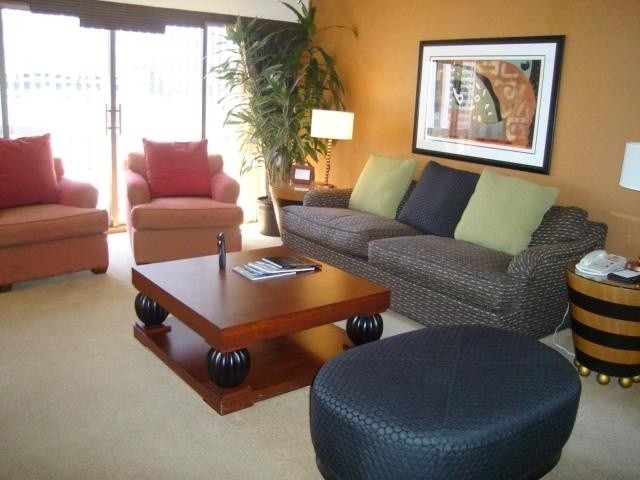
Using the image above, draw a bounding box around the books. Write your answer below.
[232,254,322,283]
[607,268,640,283]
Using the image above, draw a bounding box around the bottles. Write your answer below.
[217,232,227,270]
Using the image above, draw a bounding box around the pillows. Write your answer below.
[142,137,211,200]
[0,131,59,209]
[348,153,560,255]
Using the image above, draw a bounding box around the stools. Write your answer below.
[308,323,582,480]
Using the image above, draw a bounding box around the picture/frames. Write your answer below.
[291,165,314,185]
[410,35,569,176]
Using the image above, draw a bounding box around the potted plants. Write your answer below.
[200,0,360,236]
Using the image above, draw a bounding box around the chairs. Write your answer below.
[0,157,109,293]
[123,152,243,265]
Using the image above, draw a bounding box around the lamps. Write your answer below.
[310,109,355,191]
[619,141,640,272]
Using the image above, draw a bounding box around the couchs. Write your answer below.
[277,178,608,340]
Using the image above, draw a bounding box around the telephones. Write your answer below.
[575,249,628,276]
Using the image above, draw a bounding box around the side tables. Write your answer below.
[564,258,640,388]
[269,181,334,242]
